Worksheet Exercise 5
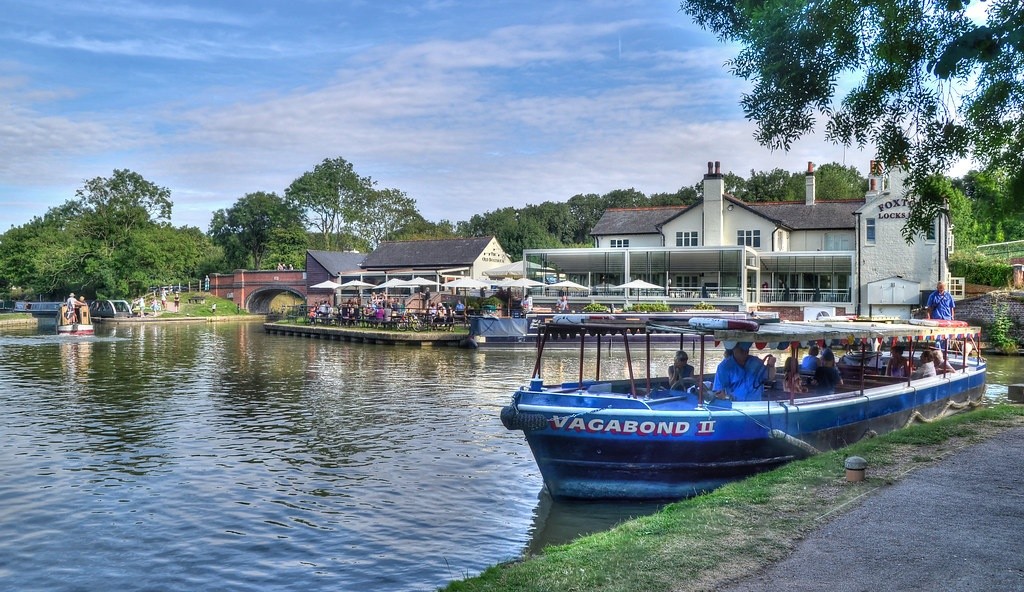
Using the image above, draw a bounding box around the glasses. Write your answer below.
[679,357,688,362]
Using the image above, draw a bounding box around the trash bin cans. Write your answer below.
[481,305,496,312]
[512,311,522,318]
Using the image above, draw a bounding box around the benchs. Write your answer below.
[629,364,953,401]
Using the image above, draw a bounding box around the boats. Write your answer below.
[14,300,134,336]
[500,313,986,500]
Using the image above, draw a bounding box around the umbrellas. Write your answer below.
[310,260,593,297]
[613,279,664,300]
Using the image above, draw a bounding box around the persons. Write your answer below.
[926,280,956,350]
[277,263,294,270]
[419,287,465,332]
[66,293,87,323]
[556,295,568,313]
[174,293,180,312]
[362,290,405,329]
[151,299,158,315]
[308,300,336,325]
[712,342,777,401]
[520,294,529,309]
[337,295,359,326]
[667,350,695,392]
[884,346,956,381]
[161,288,167,310]
[782,346,843,395]
[138,297,145,317]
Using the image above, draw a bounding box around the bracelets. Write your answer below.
[768,364,774,367]
